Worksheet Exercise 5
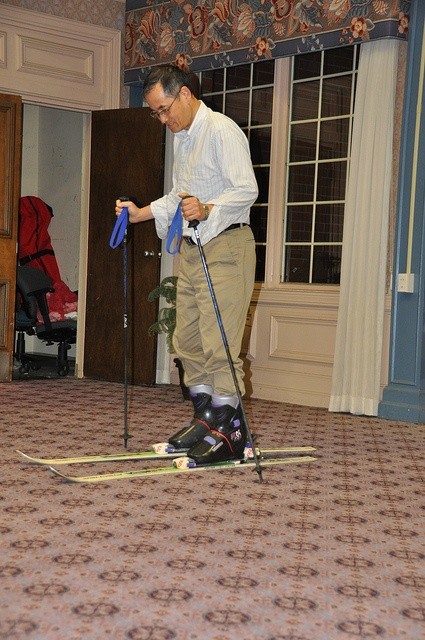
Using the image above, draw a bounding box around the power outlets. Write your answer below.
[397,273,414,293]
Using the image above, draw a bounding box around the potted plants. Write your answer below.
[148,276,190,400]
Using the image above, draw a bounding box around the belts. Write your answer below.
[182,222,247,247]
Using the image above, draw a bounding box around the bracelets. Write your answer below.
[203,201,210,221]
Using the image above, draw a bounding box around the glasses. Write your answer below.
[150,90,180,120]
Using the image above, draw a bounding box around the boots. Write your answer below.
[187,395,246,465]
[168,385,219,448]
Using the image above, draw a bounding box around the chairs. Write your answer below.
[14,266,77,377]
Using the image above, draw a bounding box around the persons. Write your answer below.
[114,64,261,461]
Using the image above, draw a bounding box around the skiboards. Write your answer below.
[15,447,319,482]
